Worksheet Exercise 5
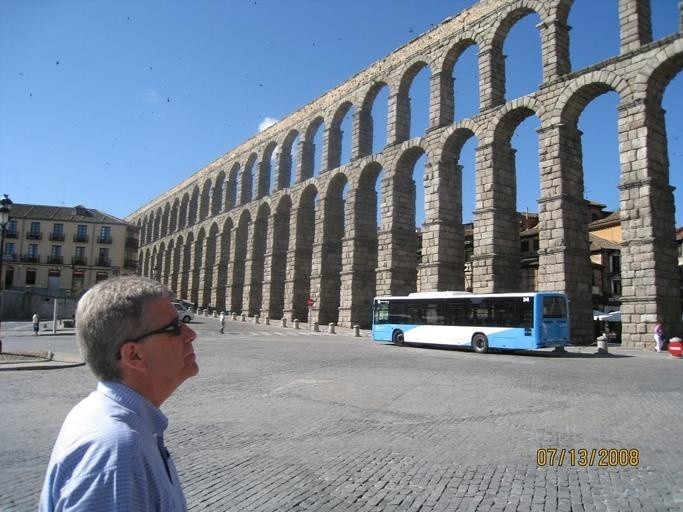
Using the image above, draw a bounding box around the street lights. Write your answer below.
[0,194,12,270]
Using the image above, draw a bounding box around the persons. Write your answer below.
[219,311,226,334]
[32,312,40,335]
[38,274,199,512]
[654,320,666,352]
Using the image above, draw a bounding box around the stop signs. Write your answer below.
[306,299,314,306]
[668,343,683,356]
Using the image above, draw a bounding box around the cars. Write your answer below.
[170,302,194,323]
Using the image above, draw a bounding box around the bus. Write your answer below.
[371,291,572,354]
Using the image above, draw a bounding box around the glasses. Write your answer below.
[114,317,178,358]
